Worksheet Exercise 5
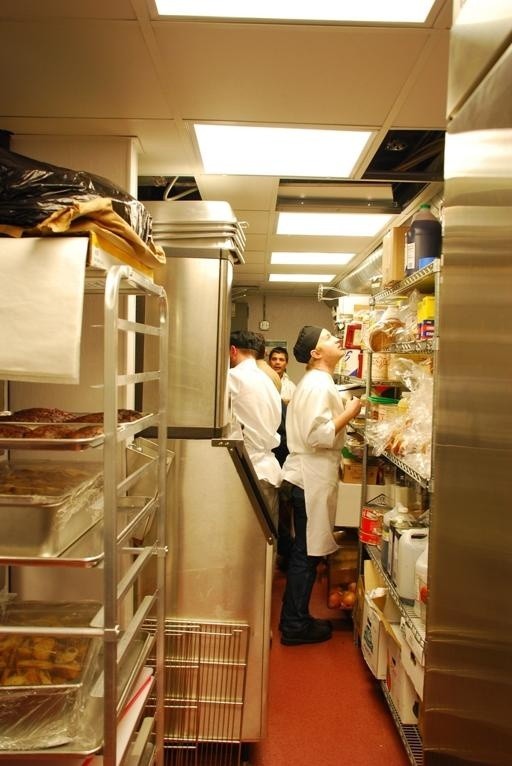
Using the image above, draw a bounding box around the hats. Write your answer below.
[231,331,265,360]
[293,325,322,364]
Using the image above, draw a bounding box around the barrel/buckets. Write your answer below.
[413,542,429,617]
[405,204,442,275]
[396,527,429,602]
[379,502,409,568]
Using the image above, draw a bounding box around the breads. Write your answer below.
[0,407,142,439]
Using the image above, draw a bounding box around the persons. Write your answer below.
[227,325,363,644]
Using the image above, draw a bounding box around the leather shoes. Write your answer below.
[279,615,332,645]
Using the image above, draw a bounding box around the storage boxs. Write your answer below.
[350,559,425,726]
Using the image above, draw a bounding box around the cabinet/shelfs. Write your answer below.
[335,259,441,723]
[1,266,171,766]
